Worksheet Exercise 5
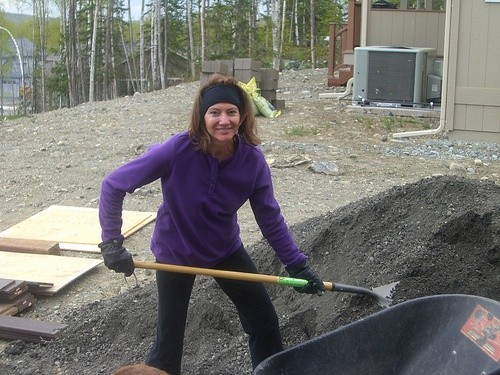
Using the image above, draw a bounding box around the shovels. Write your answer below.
[133,261,400,308]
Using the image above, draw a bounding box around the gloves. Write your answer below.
[98,236,135,277]
[285,260,325,296]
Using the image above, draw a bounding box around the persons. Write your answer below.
[99,73,326,375]
